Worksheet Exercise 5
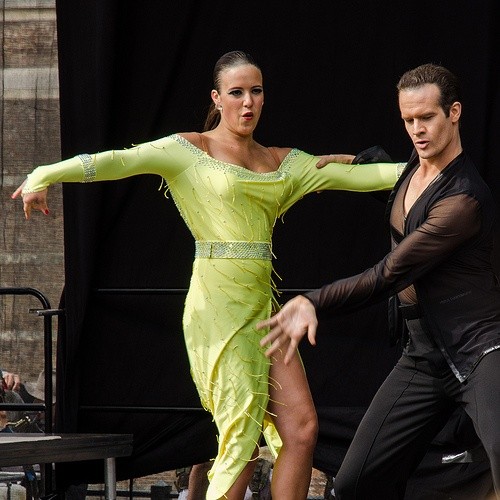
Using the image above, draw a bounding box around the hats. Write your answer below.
[23,367,57,404]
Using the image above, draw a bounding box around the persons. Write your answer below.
[11,48,407,500]
[179,459,215,500]
[258,61,500,500]
[0,370,89,500]
